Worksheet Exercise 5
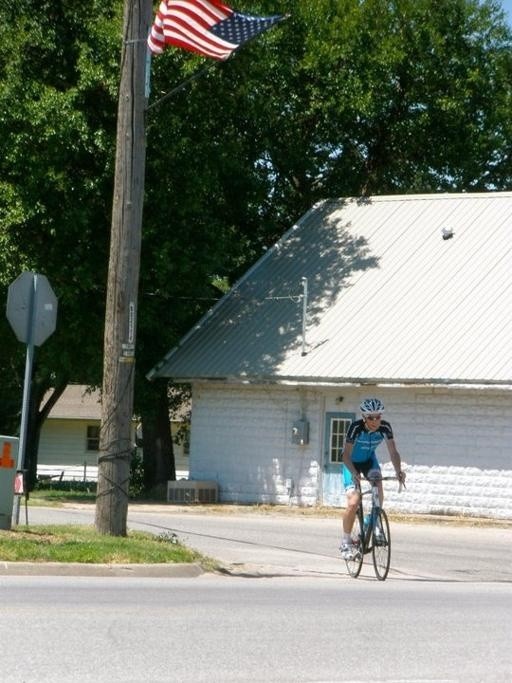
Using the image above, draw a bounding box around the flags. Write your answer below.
[146,0,295,64]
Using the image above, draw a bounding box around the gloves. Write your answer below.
[396,471,406,483]
[352,472,361,484]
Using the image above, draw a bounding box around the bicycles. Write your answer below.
[345,477,406,580]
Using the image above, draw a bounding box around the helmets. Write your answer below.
[359,398,385,414]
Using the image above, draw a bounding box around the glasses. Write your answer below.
[367,416,381,420]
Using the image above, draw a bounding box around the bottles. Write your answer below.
[360,513,374,535]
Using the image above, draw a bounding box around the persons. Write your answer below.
[340,398,406,552]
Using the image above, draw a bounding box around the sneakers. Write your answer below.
[339,543,354,560]
[374,526,386,546]
